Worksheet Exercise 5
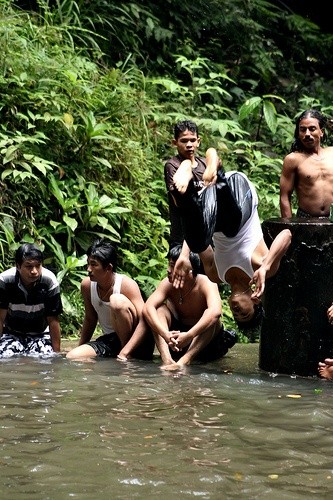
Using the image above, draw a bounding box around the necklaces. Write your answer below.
[233,287,250,297]
[98,281,115,305]
[177,280,197,305]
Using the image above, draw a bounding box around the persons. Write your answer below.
[164,108,333,387]
[64,239,157,365]
[0,243,63,355]
[143,243,227,379]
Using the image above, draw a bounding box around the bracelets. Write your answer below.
[117,355,128,361]
